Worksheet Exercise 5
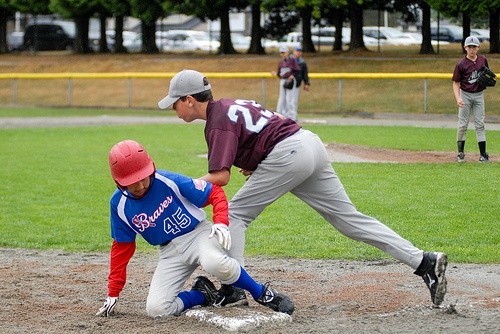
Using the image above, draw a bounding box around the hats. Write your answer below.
[157,69,211,110]
[280,46,289,53]
[294,45,302,51]
[465,36,480,47]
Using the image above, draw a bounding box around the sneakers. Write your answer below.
[217,285,248,307]
[192,276,217,307]
[255,282,295,316]
[413,252,448,305]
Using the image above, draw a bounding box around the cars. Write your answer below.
[8,19,491,52]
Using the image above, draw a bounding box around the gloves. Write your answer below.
[96,296,119,316]
[209,223,232,250]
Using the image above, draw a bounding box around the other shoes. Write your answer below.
[479,152,491,162]
[457,152,466,164]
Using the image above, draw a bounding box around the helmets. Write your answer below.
[108,140,155,192]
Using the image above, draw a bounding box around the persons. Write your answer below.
[451,36,497,163]
[95,139,295,319]
[158,68,448,308]
[273,46,311,121]
